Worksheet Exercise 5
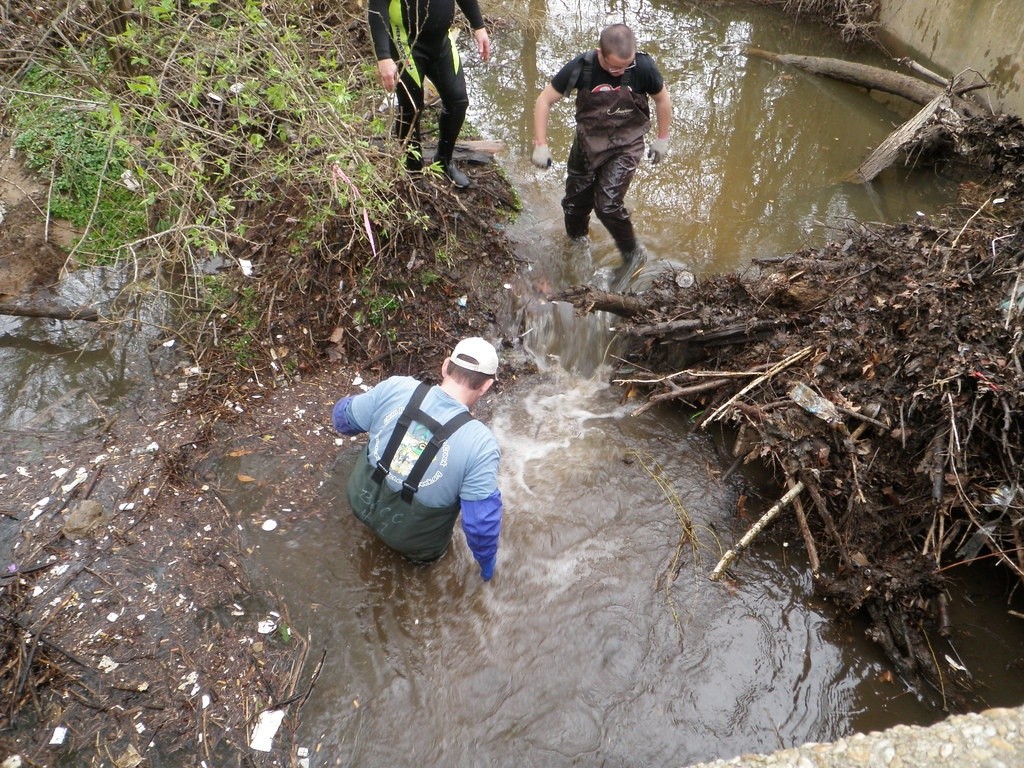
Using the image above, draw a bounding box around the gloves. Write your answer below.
[648,138,670,164]
[460,489,502,581]
[332,393,361,437]
[531,144,553,169]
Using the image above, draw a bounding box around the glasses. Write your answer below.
[602,54,636,73]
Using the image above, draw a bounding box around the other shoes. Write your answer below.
[613,250,646,289]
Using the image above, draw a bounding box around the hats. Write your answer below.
[450,336,498,374]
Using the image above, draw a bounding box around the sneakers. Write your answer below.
[401,140,424,173]
[432,153,470,188]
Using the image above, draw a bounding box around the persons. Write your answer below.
[531,25,672,261]
[332,336,502,584]
[366,0,489,188]
